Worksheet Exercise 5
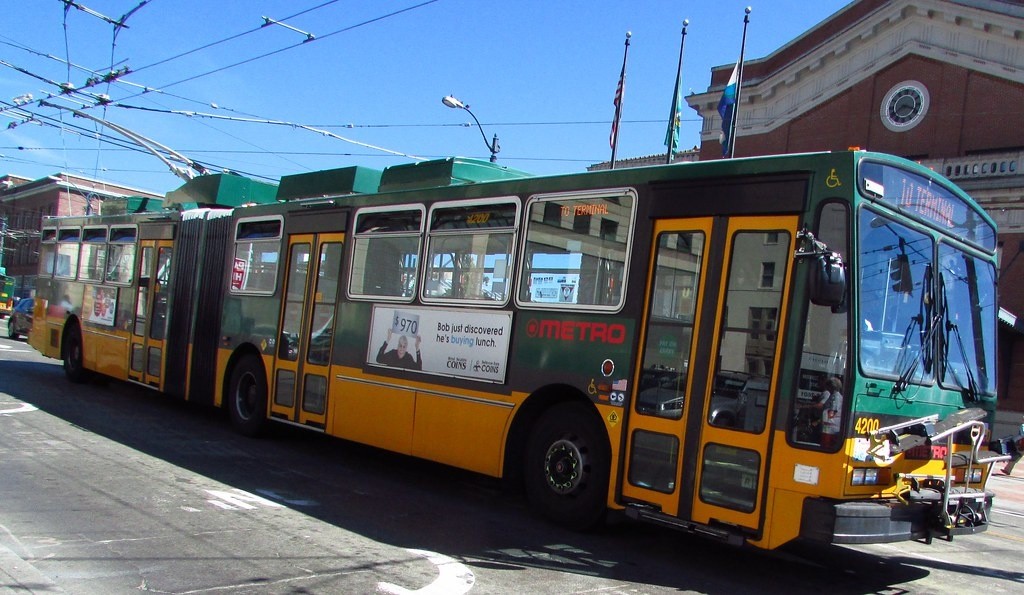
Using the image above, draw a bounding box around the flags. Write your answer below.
[717,59,741,157]
[663,74,682,161]
[609,65,626,148]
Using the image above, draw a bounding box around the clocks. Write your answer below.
[880,79,930,132]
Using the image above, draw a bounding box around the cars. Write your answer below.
[8,298,35,340]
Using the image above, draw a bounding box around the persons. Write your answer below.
[1000,416,1024,475]
[794,378,843,450]
[376,328,422,371]
[59,294,73,312]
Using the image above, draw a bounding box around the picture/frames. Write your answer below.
[367,304,514,385]
[80,284,119,327]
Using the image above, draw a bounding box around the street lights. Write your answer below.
[442,94,502,163]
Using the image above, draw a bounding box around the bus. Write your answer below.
[0,272,16,319]
[29,148,1014,557]
